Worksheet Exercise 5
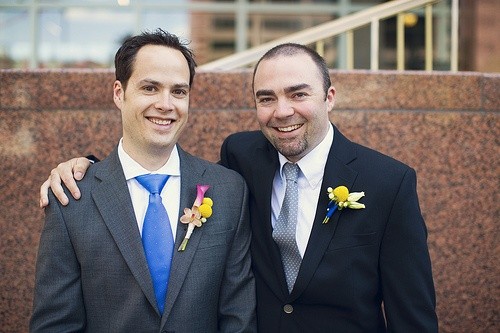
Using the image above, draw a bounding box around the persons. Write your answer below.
[40,43,439,332]
[30,26,257,332]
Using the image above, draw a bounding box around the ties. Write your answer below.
[271,162,303,293]
[133,174,176,315]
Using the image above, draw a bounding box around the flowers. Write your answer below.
[323,185,366,224]
[177,183,214,251]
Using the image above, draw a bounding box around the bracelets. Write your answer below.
[88,159,94,164]
